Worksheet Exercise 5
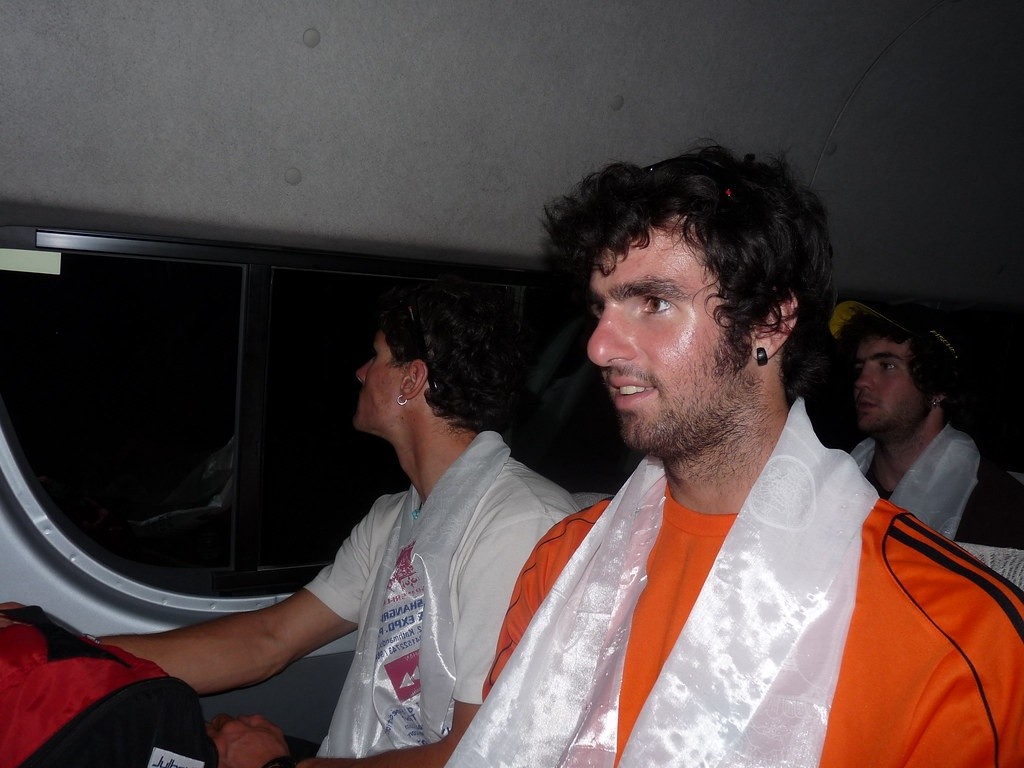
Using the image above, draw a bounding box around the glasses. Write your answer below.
[397,272,450,405]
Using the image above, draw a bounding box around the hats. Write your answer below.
[830,300,963,377]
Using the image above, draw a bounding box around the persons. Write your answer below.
[830,299,1024,551]
[0,278,582,768]
[440,146,1024,767]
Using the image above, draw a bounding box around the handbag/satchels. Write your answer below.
[0,606,219,768]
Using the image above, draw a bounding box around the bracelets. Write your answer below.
[82,633,100,645]
[261,756,303,768]
